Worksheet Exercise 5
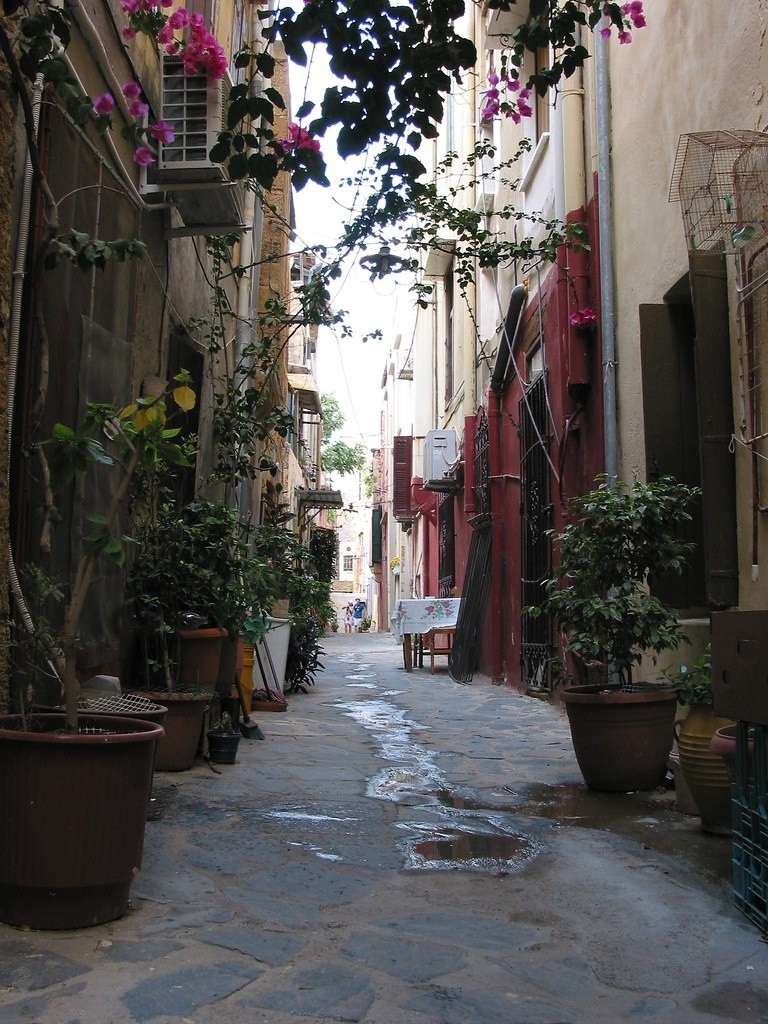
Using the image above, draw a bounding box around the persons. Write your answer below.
[342,598,364,633]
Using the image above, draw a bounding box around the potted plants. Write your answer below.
[0,362,197,941]
[0,492,306,778]
[667,638,740,839]
[519,468,703,790]
[331,622,338,632]
[708,722,755,785]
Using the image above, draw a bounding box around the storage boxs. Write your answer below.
[709,609,768,935]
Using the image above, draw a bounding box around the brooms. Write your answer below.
[233,668,268,740]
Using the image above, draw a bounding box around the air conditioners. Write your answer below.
[156,39,245,228]
[422,427,457,488]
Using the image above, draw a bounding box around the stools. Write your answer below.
[429,627,455,675]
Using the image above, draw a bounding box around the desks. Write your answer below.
[388,597,462,672]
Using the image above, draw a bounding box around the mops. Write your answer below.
[252,641,288,704]
